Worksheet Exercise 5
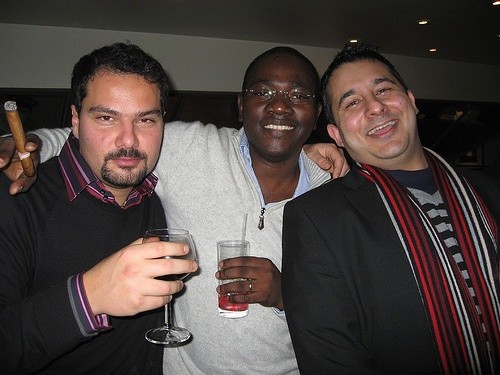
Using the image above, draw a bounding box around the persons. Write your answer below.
[0,41,351,375]
[280,40,500,375]
[0,46,333,375]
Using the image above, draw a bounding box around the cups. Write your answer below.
[216,239,250,318]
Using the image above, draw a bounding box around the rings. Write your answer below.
[248,281,252,293]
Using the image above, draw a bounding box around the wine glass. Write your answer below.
[142,229,197,344]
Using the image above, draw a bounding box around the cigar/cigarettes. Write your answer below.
[4,100,36,178]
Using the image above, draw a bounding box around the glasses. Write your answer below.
[242,86,316,104]
